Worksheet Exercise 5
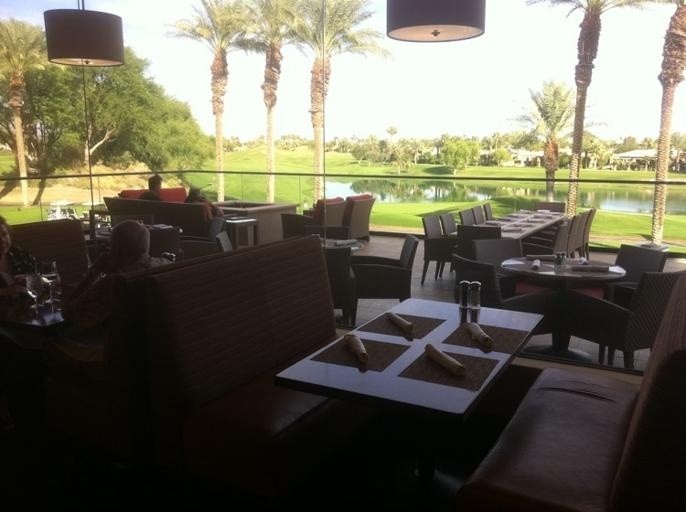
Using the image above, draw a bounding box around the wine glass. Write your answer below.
[40,272,59,305]
[25,271,44,310]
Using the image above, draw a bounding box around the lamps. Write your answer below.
[382,0,488,44]
[40,0,127,69]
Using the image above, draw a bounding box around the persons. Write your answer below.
[70,219,171,315]
[183,187,224,218]
[0,216,41,305]
[138,175,162,201]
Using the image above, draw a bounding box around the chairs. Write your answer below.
[0,184,686,510]
